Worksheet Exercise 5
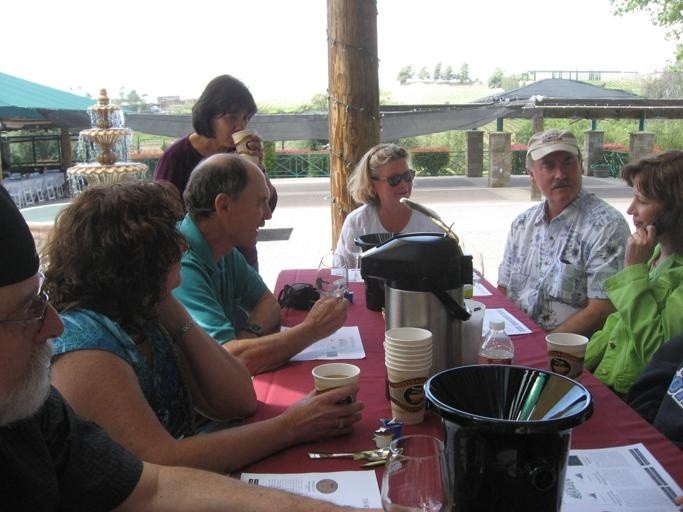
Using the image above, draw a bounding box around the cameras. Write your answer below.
[282,282,320,312]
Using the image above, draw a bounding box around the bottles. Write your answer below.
[463,284,473,299]
[43,166,47,175]
[476,319,515,368]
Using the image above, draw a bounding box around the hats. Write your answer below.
[526,129,578,160]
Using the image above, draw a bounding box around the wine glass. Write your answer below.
[344,227,368,282]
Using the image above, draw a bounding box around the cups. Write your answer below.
[310,361,361,429]
[378,433,445,512]
[470,250,485,287]
[314,253,348,300]
[382,326,434,426]
[545,332,589,385]
[231,128,261,167]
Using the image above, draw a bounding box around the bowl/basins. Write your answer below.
[8,168,42,179]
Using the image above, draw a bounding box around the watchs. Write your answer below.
[240,322,268,337]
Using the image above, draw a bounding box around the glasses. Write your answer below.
[372,169,415,185]
[0,273,50,333]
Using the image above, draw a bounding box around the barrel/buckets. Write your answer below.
[421,361,595,511]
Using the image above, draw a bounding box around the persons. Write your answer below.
[331,143,448,269]
[497,127,631,339]
[40,177,365,476]
[151,73,278,274]
[582,149,683,402]
[626,334,683,512]
[0,182,428,512]
[167,151,350,378]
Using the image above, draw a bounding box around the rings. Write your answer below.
[337,417,345,429]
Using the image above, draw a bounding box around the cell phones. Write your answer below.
[642,213,666,236]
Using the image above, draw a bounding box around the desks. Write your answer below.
[229,269,682,512]
